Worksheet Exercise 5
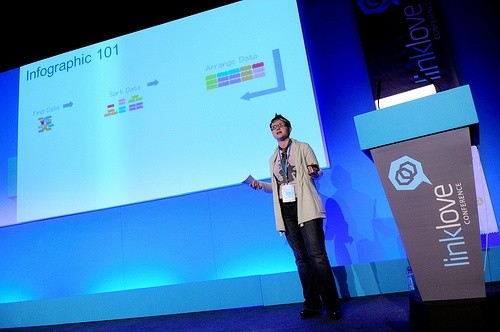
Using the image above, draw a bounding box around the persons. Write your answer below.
[249,112,342,322]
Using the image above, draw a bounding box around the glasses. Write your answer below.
[271,123,287,131]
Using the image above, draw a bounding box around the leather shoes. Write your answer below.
[300,309,321,319]
[326,307,341,320]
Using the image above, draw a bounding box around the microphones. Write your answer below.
[411,69,442,92]
[376,80,381,111]
